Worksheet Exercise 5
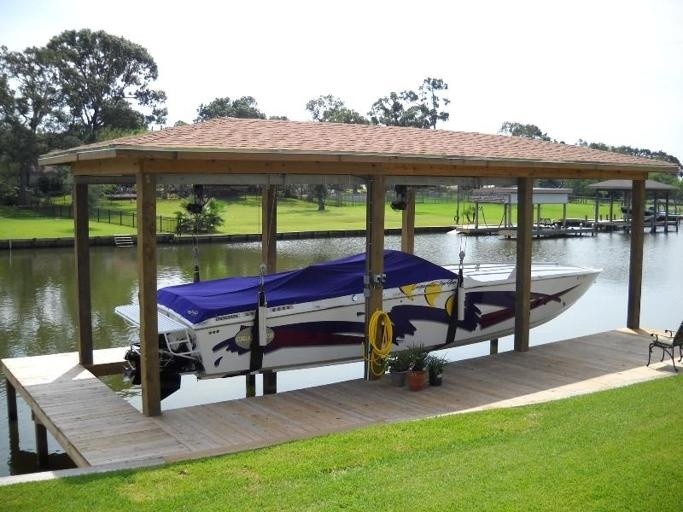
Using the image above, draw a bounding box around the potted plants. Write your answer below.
[386,341,450,392]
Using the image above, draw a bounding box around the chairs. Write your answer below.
[646,322,683,372]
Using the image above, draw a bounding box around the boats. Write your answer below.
[621,206,665,219]
[116,251,600,400]
